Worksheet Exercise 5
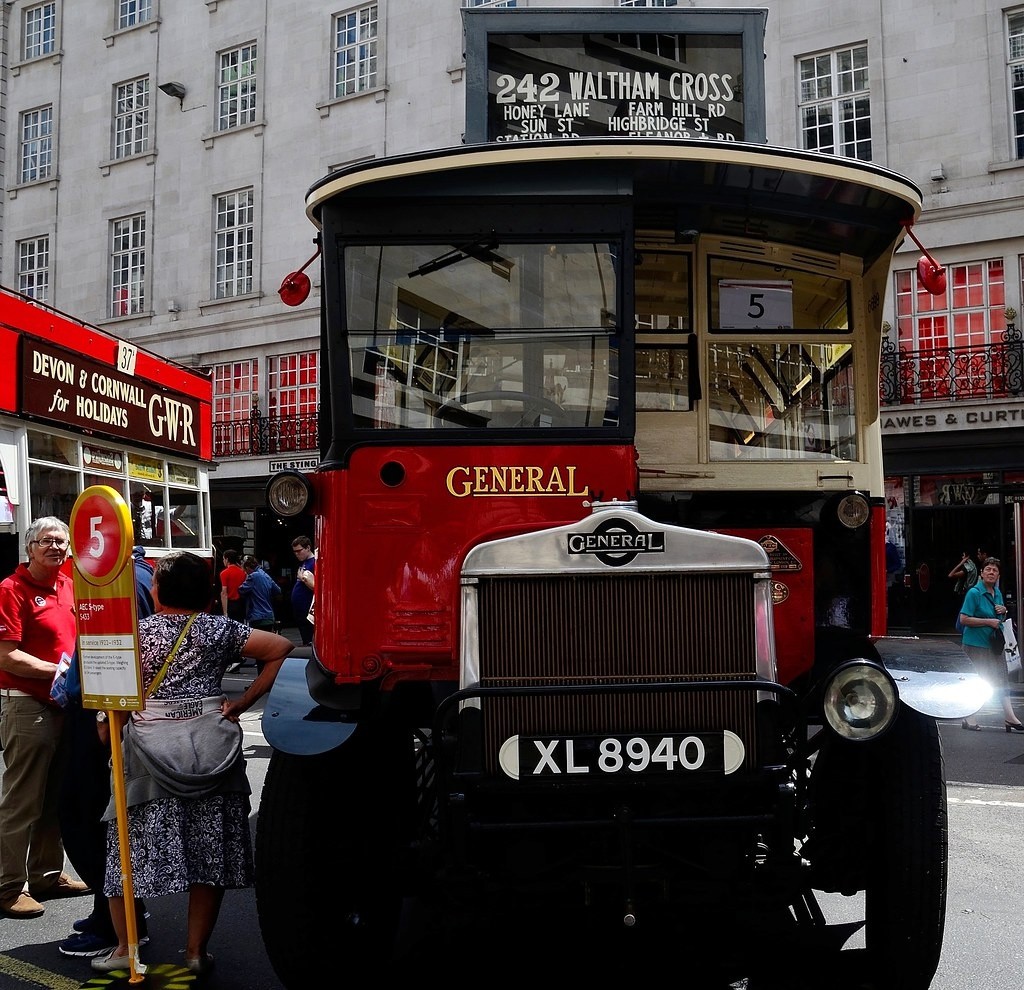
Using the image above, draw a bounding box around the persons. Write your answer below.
[0,516,315,969]
[949,547,1024,732]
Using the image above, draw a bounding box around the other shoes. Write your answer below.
[186,952,213,971]
[0,886,45,918]
[1005,720,1024,733]
[28,873,93,900]
[91,950,140,970]
[224,657,247,673]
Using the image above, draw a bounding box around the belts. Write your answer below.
[0,689,32,696]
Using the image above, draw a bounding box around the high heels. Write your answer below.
[962,718,981,731]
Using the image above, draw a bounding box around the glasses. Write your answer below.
[29,537,70,548]
[293,546,307,553]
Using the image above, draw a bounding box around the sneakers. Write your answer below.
[59,927,150,956]
[73,905,151,931]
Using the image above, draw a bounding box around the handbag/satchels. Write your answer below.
[998,618,1022,674]
[988,625,1006,656]
[956,610,965,635]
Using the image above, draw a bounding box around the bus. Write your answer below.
[0,279,216,598]
[251,131,997,990]
